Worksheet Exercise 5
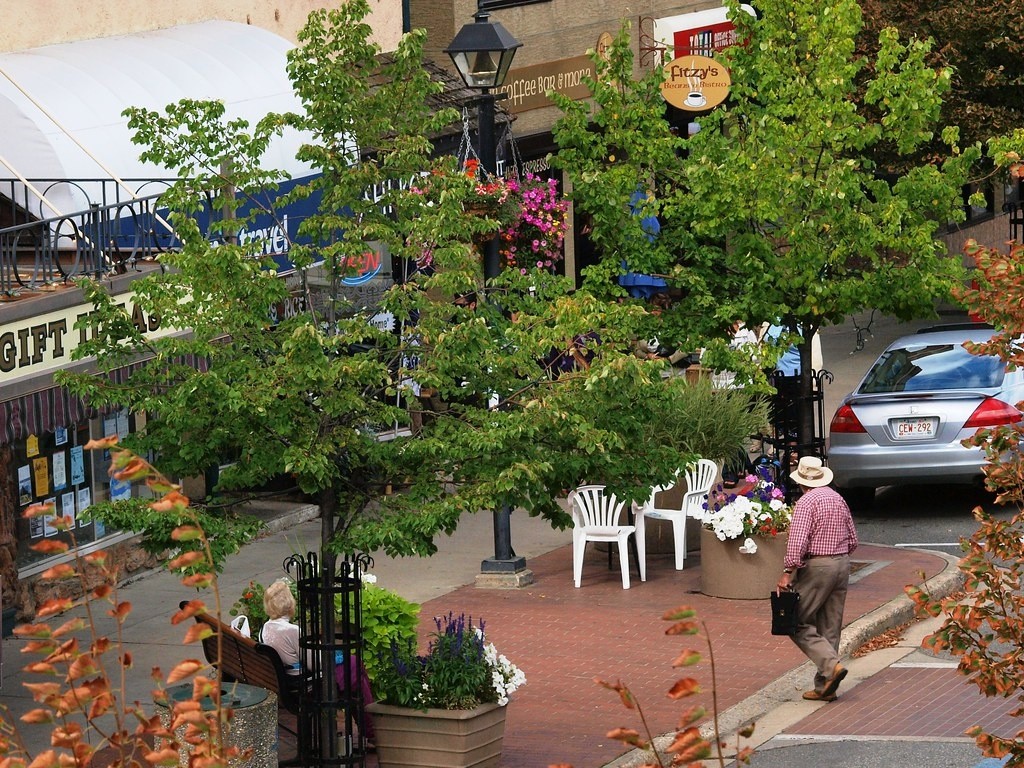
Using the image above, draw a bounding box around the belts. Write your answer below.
[804,553,847,559]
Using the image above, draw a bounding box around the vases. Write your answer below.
[365,697,507,768]
[701,527,788,600]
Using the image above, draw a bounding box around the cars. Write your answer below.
[827,322,1024,506]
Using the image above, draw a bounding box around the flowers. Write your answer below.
[378,611,527,709]
[228,533,423,684]
[701,458,794,555]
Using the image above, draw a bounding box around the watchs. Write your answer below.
[783,568,792,573]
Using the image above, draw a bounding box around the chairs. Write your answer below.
[568,485,647,590]
[644,458,717,571]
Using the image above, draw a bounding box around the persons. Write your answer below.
[777,456,858,701]
[258,582,375,753]
[699,317,823,464]
[447,290,682,509]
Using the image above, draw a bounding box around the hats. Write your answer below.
[789,456,833,487]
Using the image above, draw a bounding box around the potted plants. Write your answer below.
[594,373,774,553]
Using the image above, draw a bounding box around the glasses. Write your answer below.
[454,302,471,309]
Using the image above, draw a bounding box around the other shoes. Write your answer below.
[821,663,848,697]
[802,689,838,701]
[749,440,761,452]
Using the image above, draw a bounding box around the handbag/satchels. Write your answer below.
[231,615,250,638]
[770,592,799,635]
[293,650,344,669]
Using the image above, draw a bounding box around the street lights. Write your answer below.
[443,6,525,574]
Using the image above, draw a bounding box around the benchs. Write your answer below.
[179,601,344,749]
